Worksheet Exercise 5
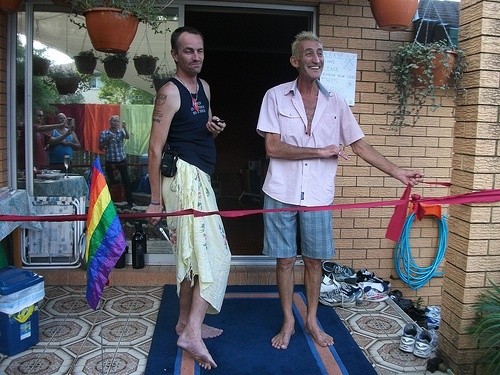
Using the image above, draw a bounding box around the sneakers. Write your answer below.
[321,260,392,308]
[398,322,437,358]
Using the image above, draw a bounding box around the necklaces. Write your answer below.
[175,70,200,113]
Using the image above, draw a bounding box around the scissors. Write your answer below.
[150,218,170,242]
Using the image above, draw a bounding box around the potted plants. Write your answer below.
[101,51,129,78]
[43,70,91,95]
[134,53,159,76]
[380,41,467,134]
[63,0,172,55]
[32,56,49,77]
[73,50,99,74]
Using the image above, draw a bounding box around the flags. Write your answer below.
[84,153,126,303]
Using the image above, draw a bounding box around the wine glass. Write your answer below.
[64,155,70,180]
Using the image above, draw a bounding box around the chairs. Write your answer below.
[20,196,87,269]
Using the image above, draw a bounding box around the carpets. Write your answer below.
[144,284,377,375]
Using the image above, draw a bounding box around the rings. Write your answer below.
[339,151,341,154]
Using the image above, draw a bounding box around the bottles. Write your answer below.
[132,221,145,269]
[115,250,125,269]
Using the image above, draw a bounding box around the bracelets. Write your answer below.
[106,140,111,145]
[149,200,162,205]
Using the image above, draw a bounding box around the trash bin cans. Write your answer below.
[0,265,44,356]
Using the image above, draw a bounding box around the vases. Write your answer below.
[368,0,418,31]
[0,0,21,14]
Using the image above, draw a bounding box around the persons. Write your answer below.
[99,115,138,207]
[256,31,425,350]
[145,26,232,371]
[18,109,81,172]
